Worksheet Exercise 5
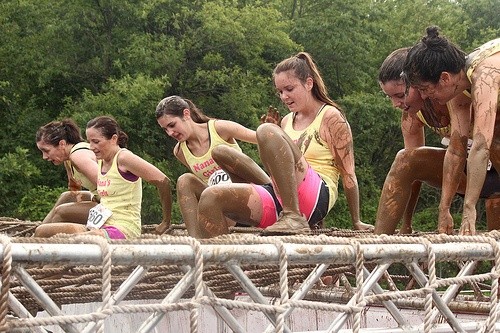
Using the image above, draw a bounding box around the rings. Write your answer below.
[465,229,469,232]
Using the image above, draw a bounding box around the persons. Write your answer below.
[33,116,172,238]
[197,52,375,238]
[156,95,283,238]
[401,25,500,236]
[373,48,500,236]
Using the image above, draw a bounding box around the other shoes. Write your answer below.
[264,210,311,236]
